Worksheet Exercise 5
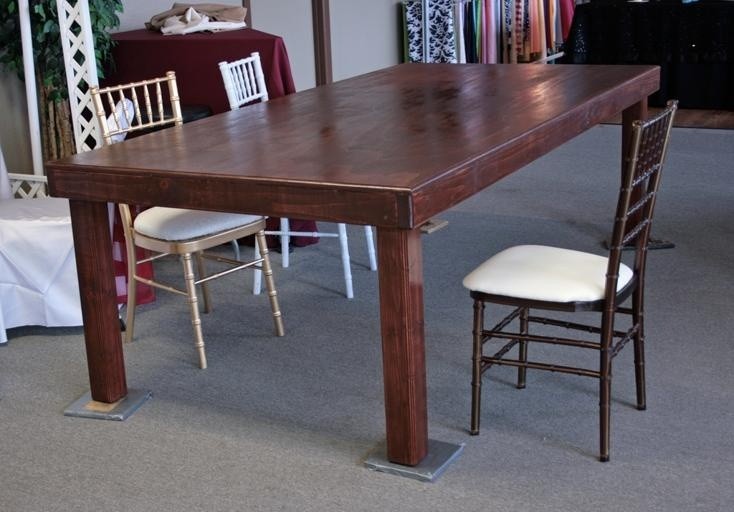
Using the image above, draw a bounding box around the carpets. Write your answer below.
[2,122,734,510]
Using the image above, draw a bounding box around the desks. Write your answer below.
[41,60,661,488]
[108,29,279,117]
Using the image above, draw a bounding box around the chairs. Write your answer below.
[458,97,680,467]
[1,150,84,343]
[90,70,285,369]
[219,51,378,299]
